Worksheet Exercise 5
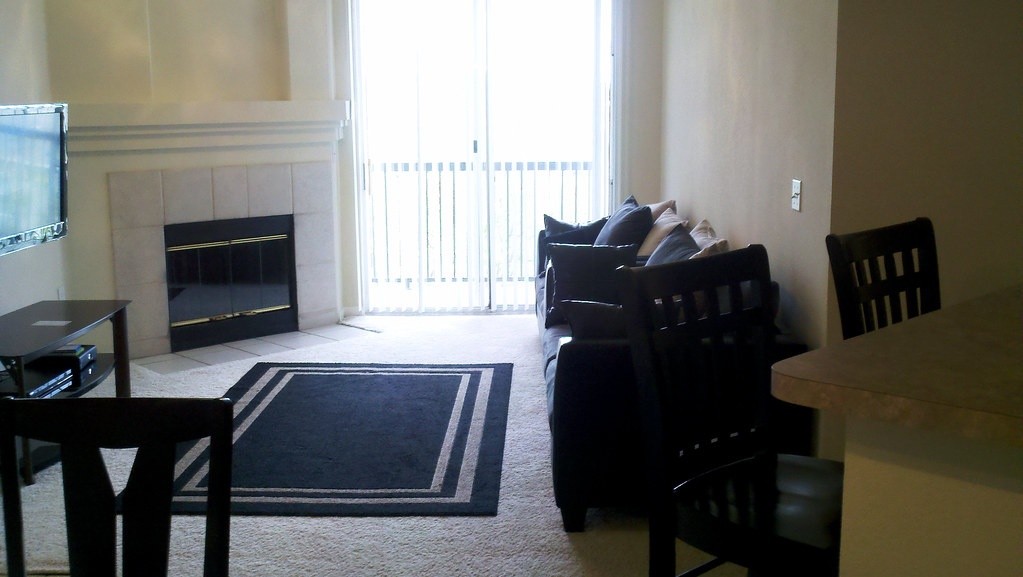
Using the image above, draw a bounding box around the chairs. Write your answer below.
[0,393,230,577]
[615,217,947,577]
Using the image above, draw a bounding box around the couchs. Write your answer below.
[536,214,814,531]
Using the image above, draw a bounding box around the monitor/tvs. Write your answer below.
[0,103,69,257]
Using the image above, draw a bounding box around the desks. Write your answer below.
[769,296,1022,575]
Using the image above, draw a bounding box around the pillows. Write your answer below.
[539,194,731,338]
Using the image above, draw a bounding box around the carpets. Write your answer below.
[113,361,514,515]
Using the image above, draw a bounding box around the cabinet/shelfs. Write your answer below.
[1,299,132,488]
